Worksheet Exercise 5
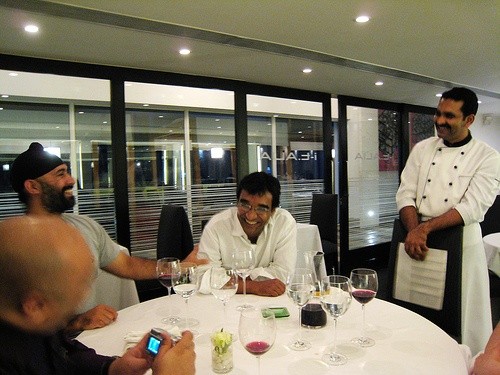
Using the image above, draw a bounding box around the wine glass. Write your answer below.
[283,267,379,367]
[239,307,277,375]
[157,247,257,342]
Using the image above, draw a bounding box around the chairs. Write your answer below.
[293,224,327,288]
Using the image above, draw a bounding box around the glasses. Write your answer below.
[237,200,273,213]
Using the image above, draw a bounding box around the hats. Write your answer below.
[9,141,63,191]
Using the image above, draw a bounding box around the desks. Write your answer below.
[482,232,500,277]
[76,287,478,375]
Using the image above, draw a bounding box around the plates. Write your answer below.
[260,308,290,320]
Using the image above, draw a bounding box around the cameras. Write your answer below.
[146,327,182,356]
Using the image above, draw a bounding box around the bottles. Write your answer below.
[301,250,327,329]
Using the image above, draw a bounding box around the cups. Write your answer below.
[210,328,233,374]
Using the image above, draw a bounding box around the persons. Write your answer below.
[189,171,296,296]
[9,142,208,338]
[471,321,500,375]
[0,215,196,375]
[386,87,500,356]
